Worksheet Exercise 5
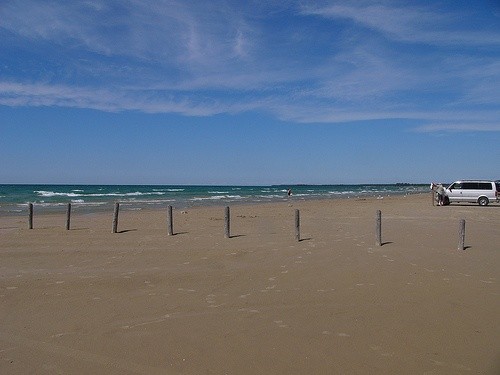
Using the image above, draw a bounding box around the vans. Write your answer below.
[435,179,498,206]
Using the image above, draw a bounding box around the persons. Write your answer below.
[436,182,444,206]
